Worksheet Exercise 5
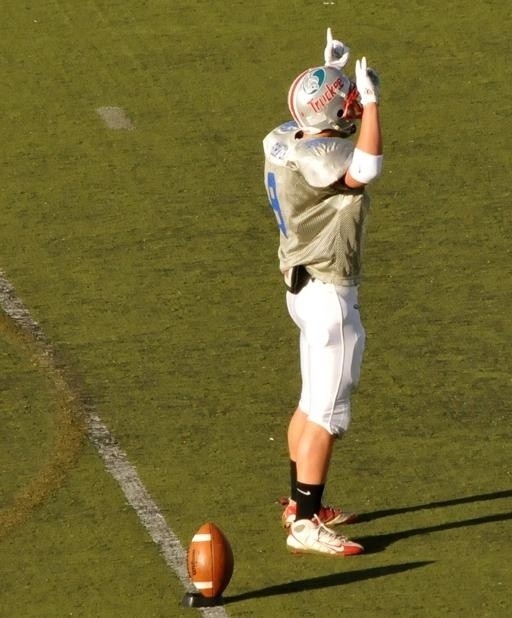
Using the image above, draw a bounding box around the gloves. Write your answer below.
[355,56,381,103]
[324,27,351,69]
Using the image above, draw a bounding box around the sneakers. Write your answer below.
[277,496,360,529]
[286,513,365,556]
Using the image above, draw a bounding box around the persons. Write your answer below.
[258,24,389,558]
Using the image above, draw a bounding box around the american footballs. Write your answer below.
[186,522,233,602]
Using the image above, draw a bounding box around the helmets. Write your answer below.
[287,66,363,134]
